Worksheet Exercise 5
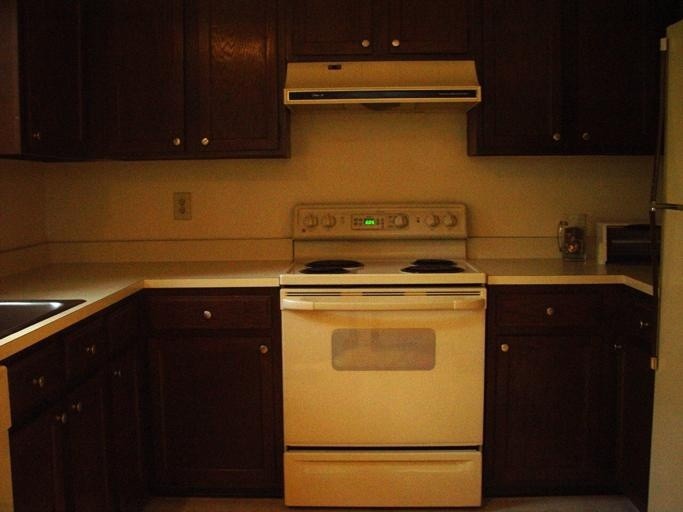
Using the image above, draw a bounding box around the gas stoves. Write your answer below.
[278,257,487,287]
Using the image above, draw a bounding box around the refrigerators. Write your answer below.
[647,18,682,512]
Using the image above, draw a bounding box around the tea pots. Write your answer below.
[555,214,587,261]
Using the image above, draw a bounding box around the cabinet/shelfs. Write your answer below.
[481,284,618,497]
[280,0,480,61]
[80,1,293,161]
[467,3,664,158]
[140,281,282,499]
[0,0,83,163]
[2,321,117,512]
[598,282,659,512]
[99,278,150,512]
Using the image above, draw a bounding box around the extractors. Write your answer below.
[283,59,481,110]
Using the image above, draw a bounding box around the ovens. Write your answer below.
[275,287,487,508]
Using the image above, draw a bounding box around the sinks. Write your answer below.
[0,299,88,339]
